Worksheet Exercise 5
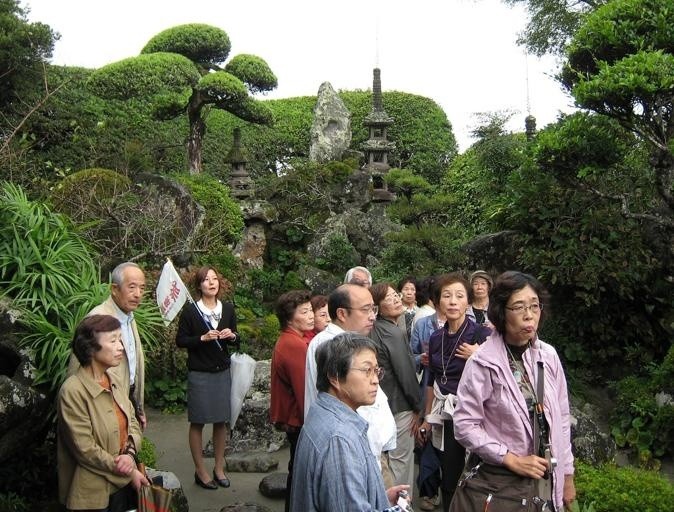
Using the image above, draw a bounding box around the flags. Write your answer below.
[155,257,189,328]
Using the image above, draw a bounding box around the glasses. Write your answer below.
[350,367,386,381]
[381,293,403,303]
[348,306,378,316]
[506,302,542,313]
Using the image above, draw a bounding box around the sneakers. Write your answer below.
[420,497,434,511]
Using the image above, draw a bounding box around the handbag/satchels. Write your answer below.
[449,453,542,512]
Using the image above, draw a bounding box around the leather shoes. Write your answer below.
[194,471,219,489]
[212,467,230,488]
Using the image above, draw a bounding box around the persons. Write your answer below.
[175,264,240,490]
[69,262,146,434]
[271,265,581,511]
[56,314,150,512]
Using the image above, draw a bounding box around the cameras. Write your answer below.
[544,448,558,474]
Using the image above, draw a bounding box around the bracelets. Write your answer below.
[128,446,136,451]
[124,450,136,459]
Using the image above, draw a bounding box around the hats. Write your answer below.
[470,270,493,294]
